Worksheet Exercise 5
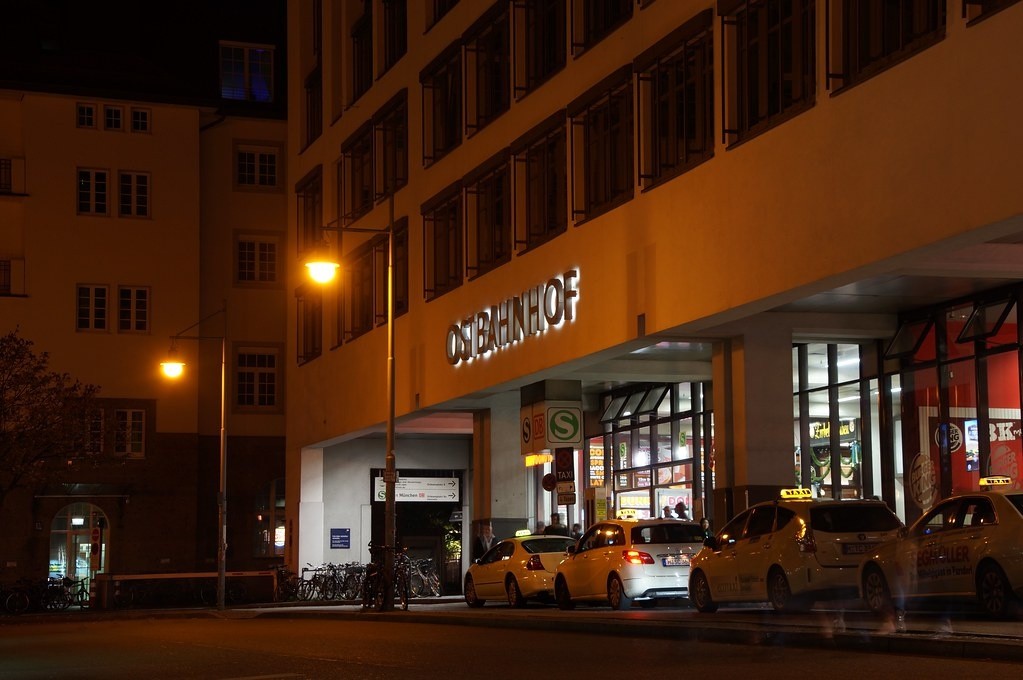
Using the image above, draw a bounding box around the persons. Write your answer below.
[532,521,547,535]
[699,517,713,542]
[662,506,674,519]
[544,512,569,538]
[472,518,501,565]
[569,523,584,540]
[674,501,689,519]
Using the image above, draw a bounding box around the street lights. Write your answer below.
[302,188,397,611]
[161,300,229,611]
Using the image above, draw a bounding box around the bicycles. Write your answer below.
[0,536,443,615]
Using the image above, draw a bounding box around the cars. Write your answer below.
[552,517,706,610]
[688,488,907,617]
[462,535,574,608]
[856,476,1023,619]
